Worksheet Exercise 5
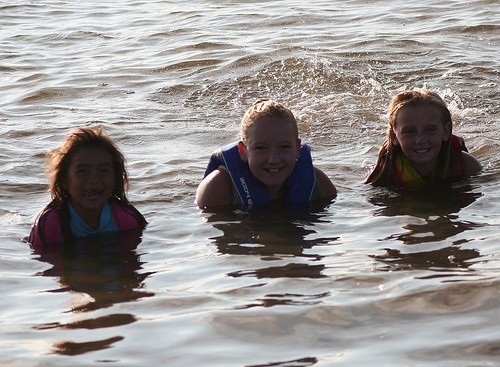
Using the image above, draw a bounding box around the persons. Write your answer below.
[26,125,148,247]
[365,90,483,188]
[194,98,338,210]
